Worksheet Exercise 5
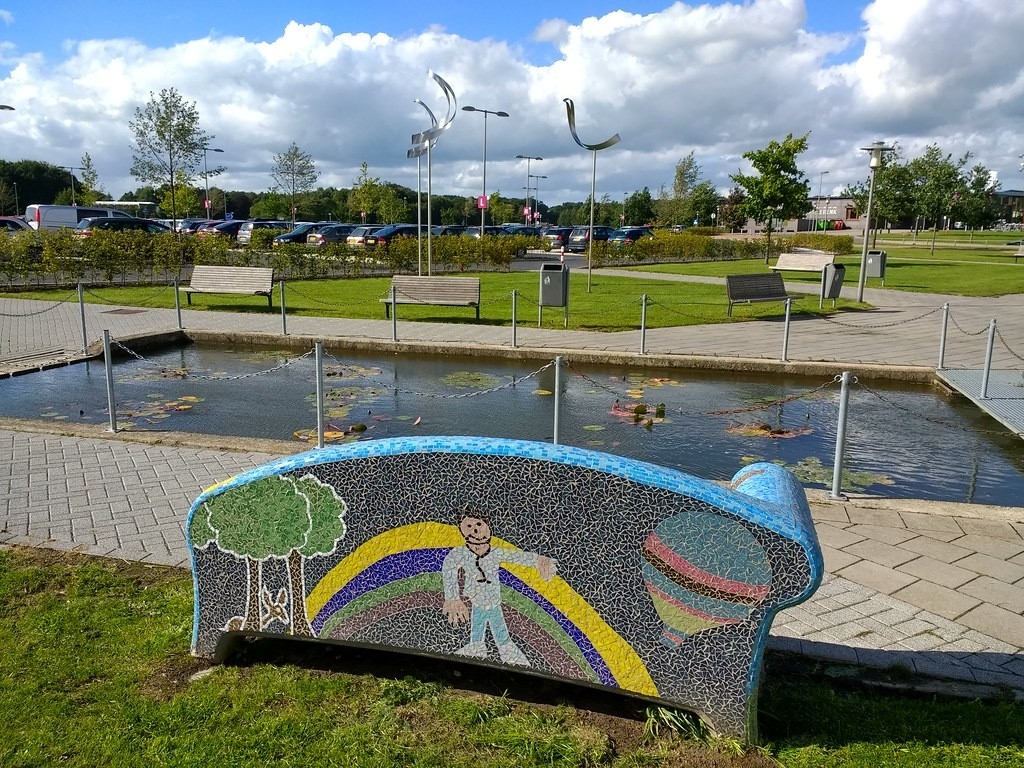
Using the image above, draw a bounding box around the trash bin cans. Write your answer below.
[817,219,829,230]
[539,263,566,307]
[866,250,883,278]
[829,219,843,230]
[824,263,846,299]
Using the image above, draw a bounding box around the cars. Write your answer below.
[540,226,573,241]
[72,217,174,236]
[237,221,298,245]
[202,220,254,243]
[307,225,359,244]
[502,227,541,250]
[346,227,385,246]
[365,224,437,253]
[542,228,576,252]
[672,225,688,231]
[144,218,228,237]
[0,217,55,256]
[607,226,661,257]
[273,223,337,245]
[460,227,528,259]
[569,226,615,254]
[429,225,467,238]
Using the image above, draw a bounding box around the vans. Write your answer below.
[25,205,133,236]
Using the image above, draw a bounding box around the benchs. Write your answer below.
[1013,246,1024,263]
[769,253,836,273]
[179,265,275,309]
[725,272,805,318]
[184,433,827,748]
[379,275,481,323]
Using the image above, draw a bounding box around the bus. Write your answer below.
[501,223,521,229]
